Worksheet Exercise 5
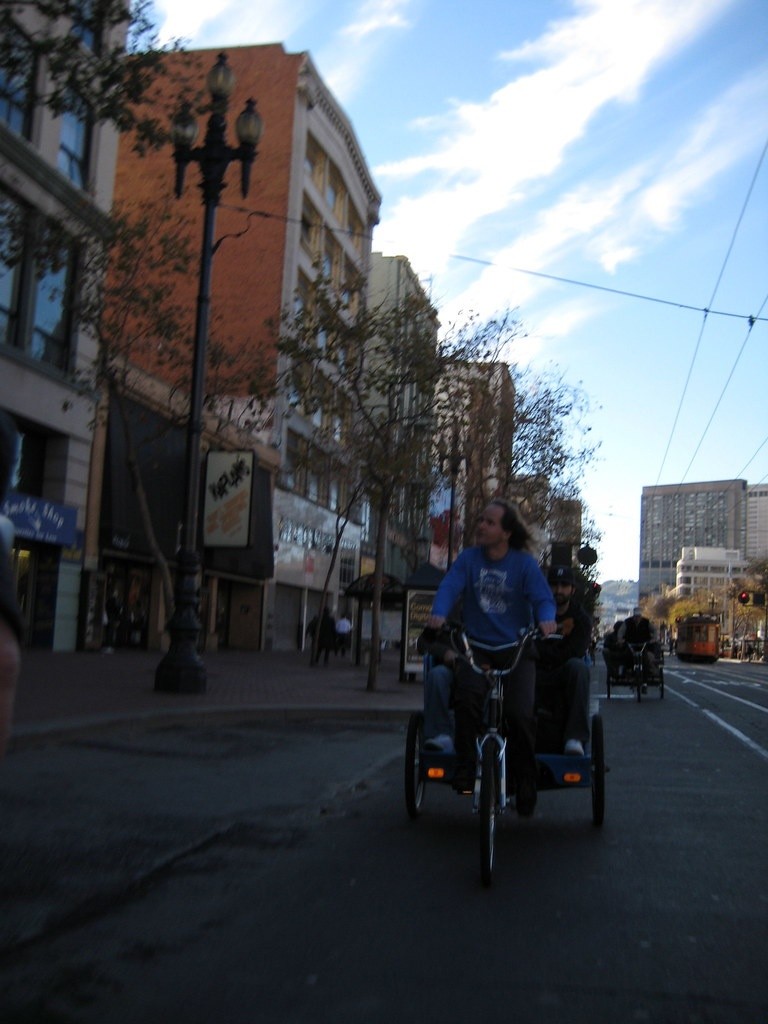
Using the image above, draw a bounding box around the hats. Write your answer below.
[549,564,573,584]
[634,608,641,617]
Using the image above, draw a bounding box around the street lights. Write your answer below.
[152,51,261,694]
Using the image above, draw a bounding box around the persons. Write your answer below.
[313,606,335,668]
[542,563,592,756]
[416,625,456,753]
[603,608,657,686]
[335,615,352,658]
[427,500,559,822]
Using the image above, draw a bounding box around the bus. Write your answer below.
[676,613,719,664]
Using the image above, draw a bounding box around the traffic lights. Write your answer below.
[737,591,750,604]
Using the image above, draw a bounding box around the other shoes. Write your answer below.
[516,781,538,817]
[422,734,454,757]
[564,738,583,755]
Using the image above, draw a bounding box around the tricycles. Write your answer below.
[606,640,664,703]
[404,619,612,888]
